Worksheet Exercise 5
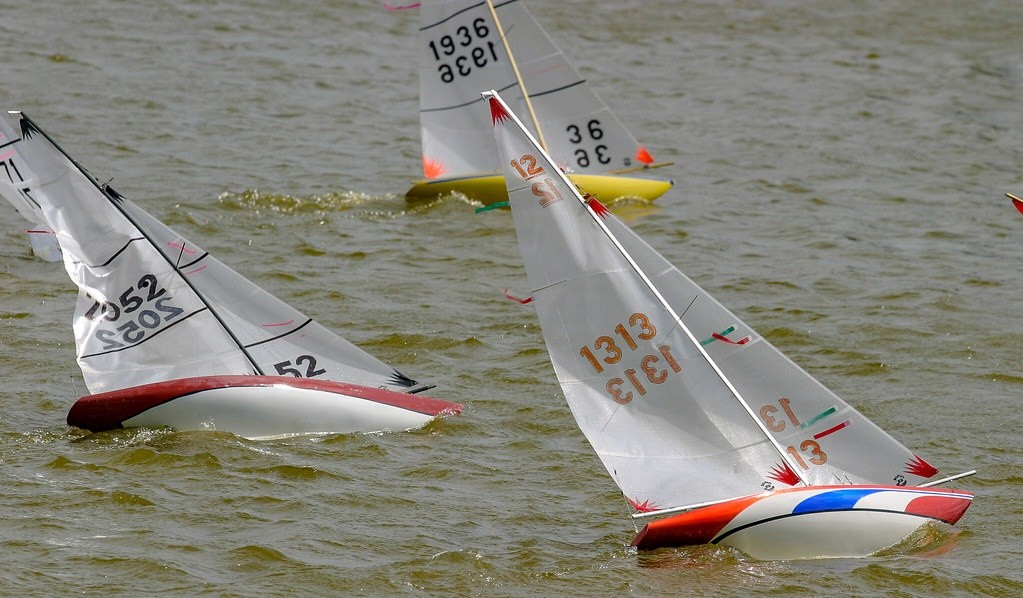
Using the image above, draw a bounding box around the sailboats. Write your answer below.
[480,90,977,559]
[403,0,677,211]
[0,108,463,441]
